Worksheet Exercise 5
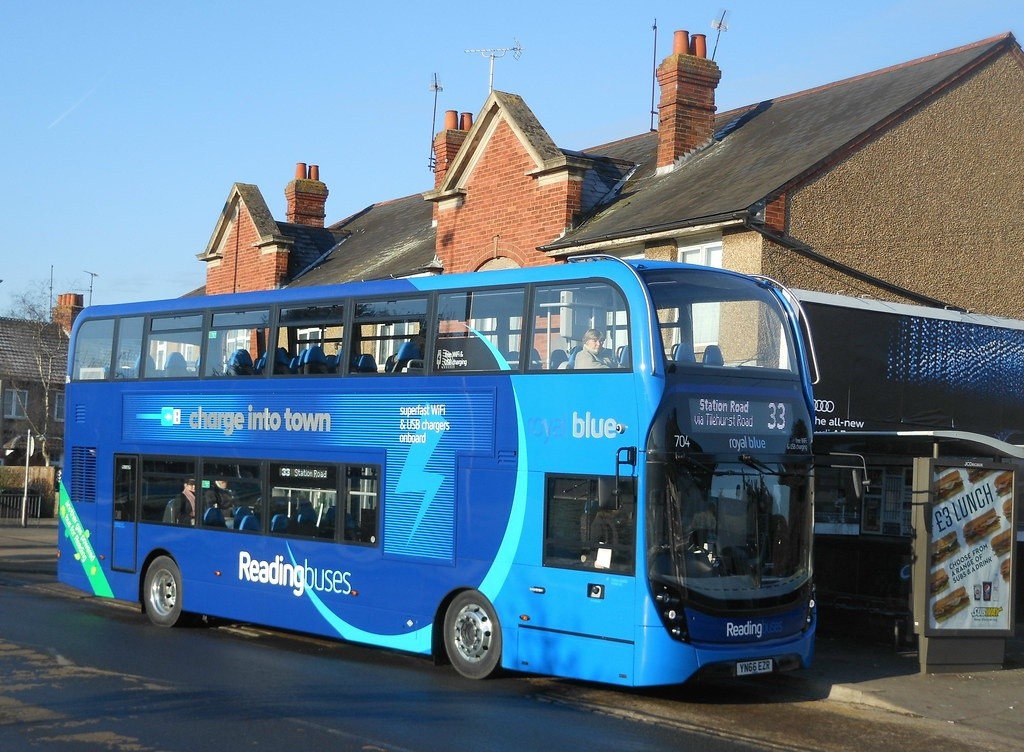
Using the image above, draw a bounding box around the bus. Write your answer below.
[59,254,872,686]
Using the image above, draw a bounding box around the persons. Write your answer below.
[691,501,718,530]
[575,328,625,369]
[174,471,243,527]
[584,492,633,562]
